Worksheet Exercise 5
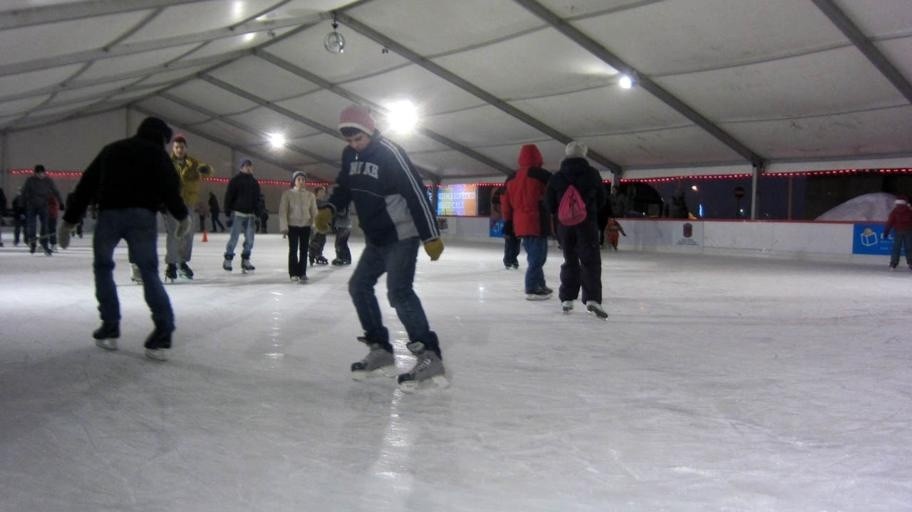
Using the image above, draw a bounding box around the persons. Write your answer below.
[279,170,353,281]
[1,164,65,254]
[208,191,226,232]
[224,158,269,270]
[883,195,912,270]
[58,115,194,360]
[489,141,627,320]
[162,136,212,280]
[312,106,445,385]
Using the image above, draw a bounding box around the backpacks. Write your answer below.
[558,185,588,227]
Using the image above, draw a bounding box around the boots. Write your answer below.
[397,332,444,385]
[351,329,394,372]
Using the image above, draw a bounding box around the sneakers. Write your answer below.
[178,262,192,275]
[525,285,553,295]
[165,263,178,278]
[241,259,255,270]
[562,299,573,311]
[223,258,233,270]
[145,330,172,351]
[585,300,609,318]
[92,322,119,340]
[290,257,350,280]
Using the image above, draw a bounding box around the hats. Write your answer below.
[173,134,186,142]
[291,171,307,184]
[566,141,588,158]
[338,104,375,137]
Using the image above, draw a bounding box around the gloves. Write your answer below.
[315,207,332,234]
[59,221,81,248]
[176,216,191,238]
[425,239,444,261]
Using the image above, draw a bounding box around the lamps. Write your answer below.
[323,19,346,53]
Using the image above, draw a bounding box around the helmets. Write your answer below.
[240,156,252,168]
